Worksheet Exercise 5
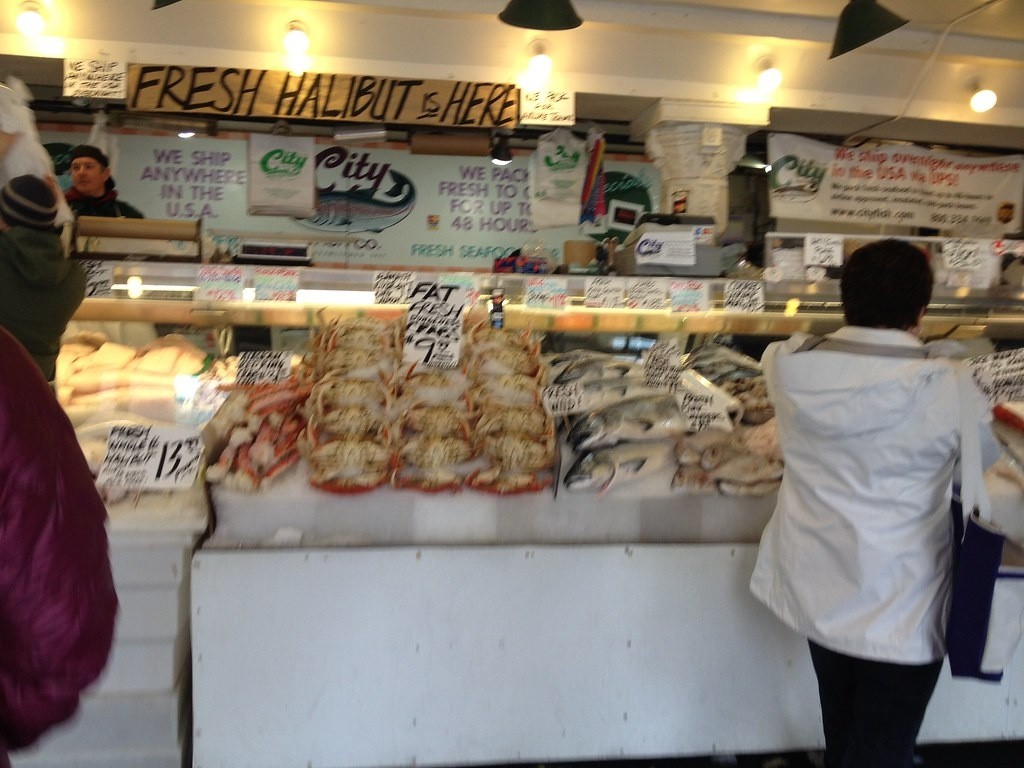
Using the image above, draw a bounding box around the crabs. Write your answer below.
[203,354,317,490]
[393,361,472,490]
[464,318,553,490]
[301,307,399,491]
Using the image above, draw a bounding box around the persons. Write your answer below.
[0,324,118,750]
[63,143,144,219]
[751,237,1002,768]
[0,127,87,384]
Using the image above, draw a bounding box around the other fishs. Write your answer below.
[547,347,670,412]
[566,393,688,453]
[562,440,682,496]
[673,426,786,495]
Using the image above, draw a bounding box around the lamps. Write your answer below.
[830,0,912,61]
[491,137,511,165]
[500,0,582,31]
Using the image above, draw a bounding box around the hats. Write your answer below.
[0,175,57,231]
[71,144,115,190]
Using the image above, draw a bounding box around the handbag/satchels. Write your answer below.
[933,358,1024,683]
